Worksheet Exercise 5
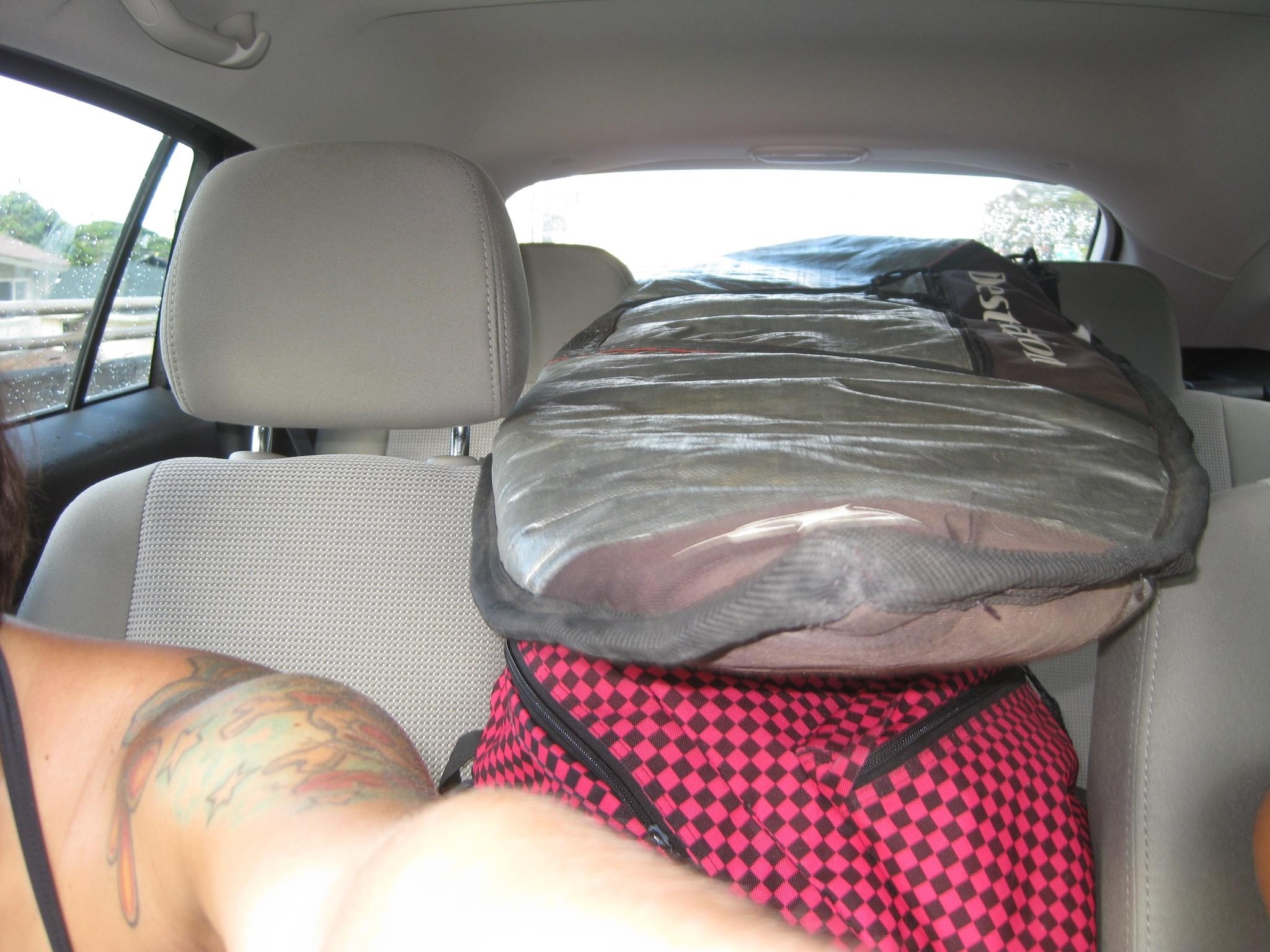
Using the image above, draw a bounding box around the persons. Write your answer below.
[0,373,848,952]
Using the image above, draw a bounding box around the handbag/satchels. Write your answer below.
[437,637,1100,952]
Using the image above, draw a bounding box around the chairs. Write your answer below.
[16,143,1270,952]
